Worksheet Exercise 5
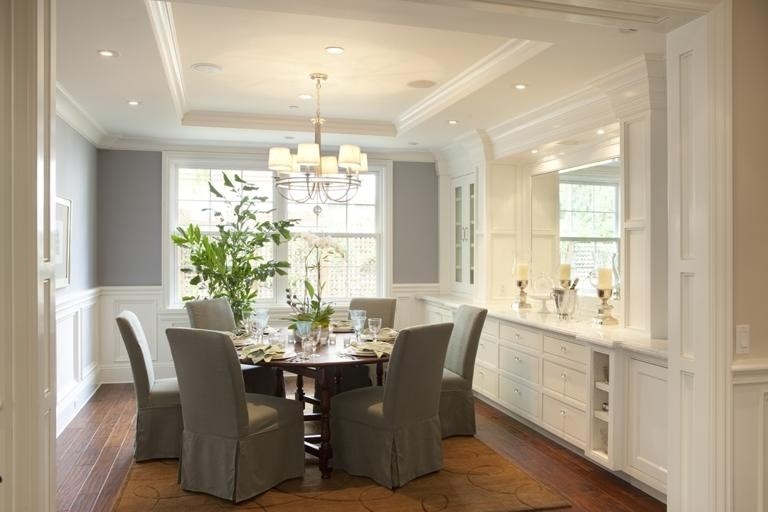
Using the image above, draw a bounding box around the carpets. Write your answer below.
[110,393,572,512]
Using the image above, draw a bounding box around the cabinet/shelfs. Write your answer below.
[436,170,522,296]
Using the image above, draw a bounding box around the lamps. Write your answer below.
[269,72,368,203]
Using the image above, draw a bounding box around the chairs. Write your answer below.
[114,310,183,463]
[165,328,305,504]
[329,323,455,492]
[312,297,397,412]
[185,295,286,398]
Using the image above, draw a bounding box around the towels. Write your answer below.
[241,342,288,364]
[232,335,251,347]
[376,327,399,343]
[351,341,394,360]
[330,320,353,333]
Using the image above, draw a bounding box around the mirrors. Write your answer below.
[527,157,622,302]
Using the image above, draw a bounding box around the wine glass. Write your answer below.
[349,310,364,347]
[528,295,553,314]
[255,311,269,347]
[307,325,321,359]
[596,288,611,309]
[516,280,527,296]
[366,318,381,347]
[558,295,577,330]
[242,312,252,336]
[297,323,310,358]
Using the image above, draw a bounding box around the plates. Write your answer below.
[266,351,297,359]
[355,351,374,354]
[340,342,392,356]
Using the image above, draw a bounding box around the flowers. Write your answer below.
[282,231,344,330]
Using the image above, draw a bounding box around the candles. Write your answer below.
[517,264,529,282]
[597,268,612,290]
[559,264,570,282]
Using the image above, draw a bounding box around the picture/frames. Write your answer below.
[55,195,72,290]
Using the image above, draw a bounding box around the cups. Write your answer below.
[343,337,350,348]
[559,280,569,288]
[329,334,335,345]
[269,327,294,351]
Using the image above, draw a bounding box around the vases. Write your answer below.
[295,321,318,343]
